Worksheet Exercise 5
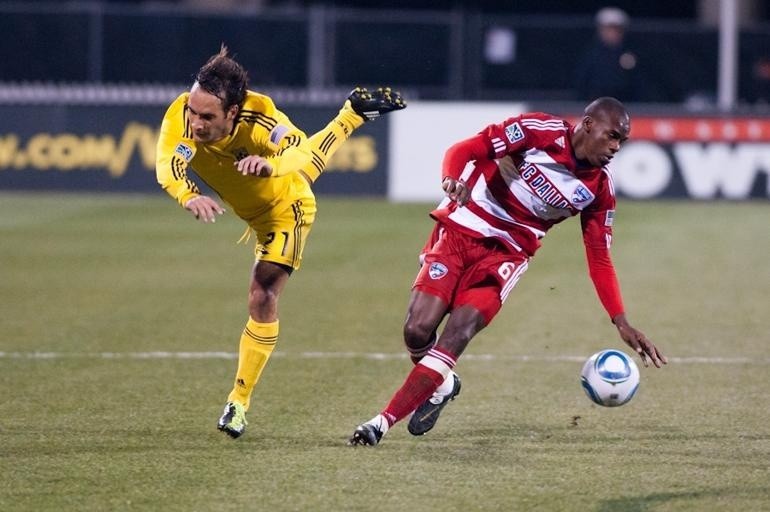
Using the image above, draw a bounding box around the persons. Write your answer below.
[154,42,409,440]
[349,97,668,447]
[574,8,663,104]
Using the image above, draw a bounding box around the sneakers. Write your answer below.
[345,409,384,447]
[407,370,462,436]
[217,400,248,438]
[347,87,409,123]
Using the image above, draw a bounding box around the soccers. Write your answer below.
[581,350,639,407]
